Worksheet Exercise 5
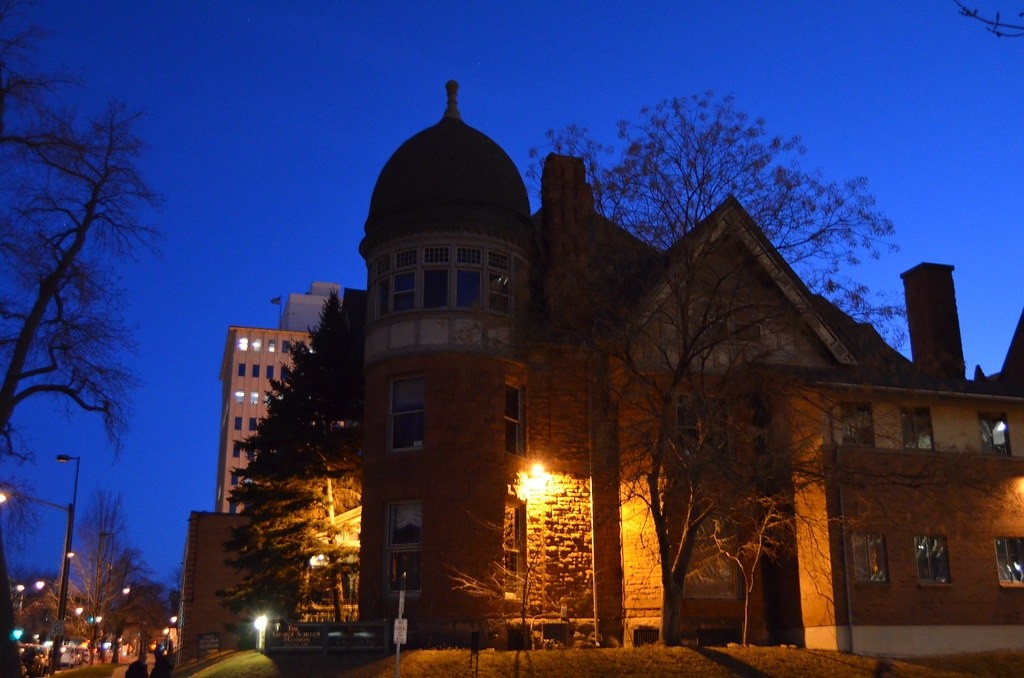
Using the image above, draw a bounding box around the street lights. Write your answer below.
[55,453,80,509]
[0,487,75,673]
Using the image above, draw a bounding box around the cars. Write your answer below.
[60,645,102,665]
[16,642,51,678]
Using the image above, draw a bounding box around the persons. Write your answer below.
[125,654,148,678]
[150,648,171,678]
